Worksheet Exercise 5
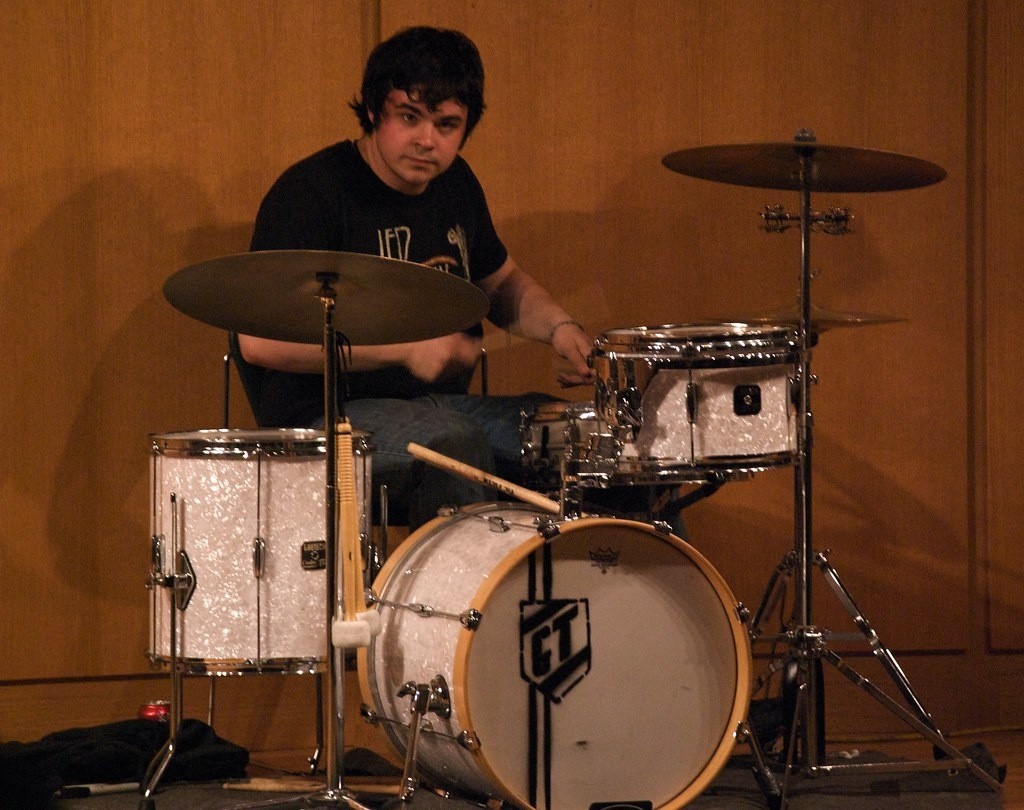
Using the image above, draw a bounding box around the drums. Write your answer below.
[143,429,374,676]
[356,498,753,810]
[599,321,804,478]
[528,400,710,489]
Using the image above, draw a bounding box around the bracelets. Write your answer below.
[552,321,583,339]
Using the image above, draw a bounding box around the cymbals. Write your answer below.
[660,142,948,193]
[742,299,912,334]
[161,249,491,346]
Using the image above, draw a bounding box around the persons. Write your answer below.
[229,25,688,543]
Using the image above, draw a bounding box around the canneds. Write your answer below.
[137,700,172,722]
